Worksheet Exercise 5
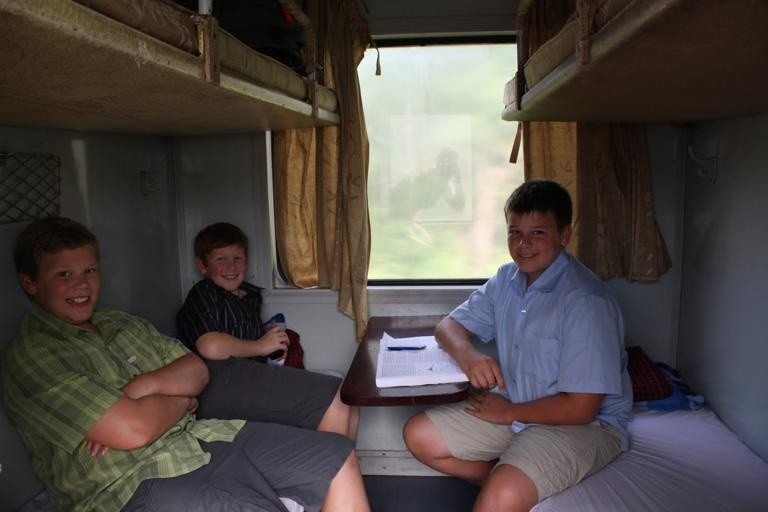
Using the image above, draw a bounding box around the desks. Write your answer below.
[340,315,500,406]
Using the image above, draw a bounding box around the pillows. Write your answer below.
[633,359,709,413]
[280,325,306,368]
[162,312,286,368]
[623,350,674,396]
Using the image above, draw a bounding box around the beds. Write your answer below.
[488,350,768,512]
[499,0,768,123]
[1,369,344,512]
[0,0,345,128]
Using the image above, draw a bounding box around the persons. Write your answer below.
[0,214,372,512]
[402,180,633,512]
[178,220,360,442]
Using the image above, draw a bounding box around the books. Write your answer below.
[375,331,470,388]
